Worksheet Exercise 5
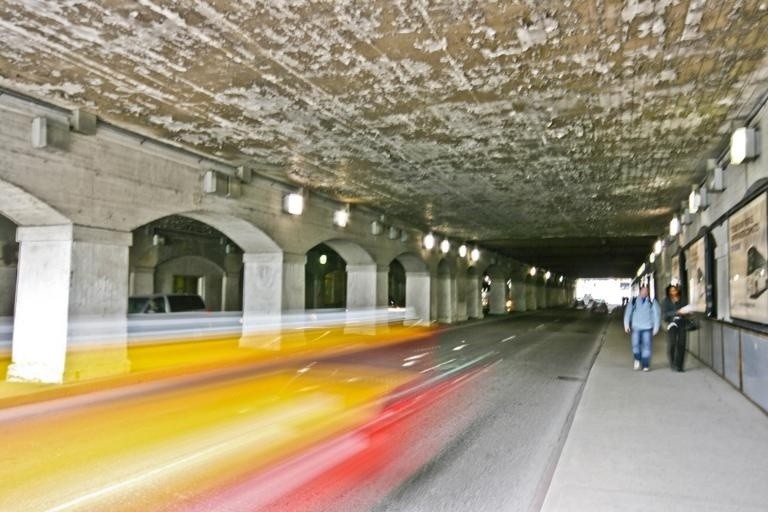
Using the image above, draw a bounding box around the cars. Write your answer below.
[591,299,608,313]
[575,298,586,310]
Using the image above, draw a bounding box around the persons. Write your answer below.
[659,285,700,373]
[622,284,662,373]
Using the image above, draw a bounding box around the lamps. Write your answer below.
[282,191,482,264]
[635,126,760,277]
[30,111,98,156]
[201,165,251,201]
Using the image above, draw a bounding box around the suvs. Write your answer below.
[128,292,208,316]
